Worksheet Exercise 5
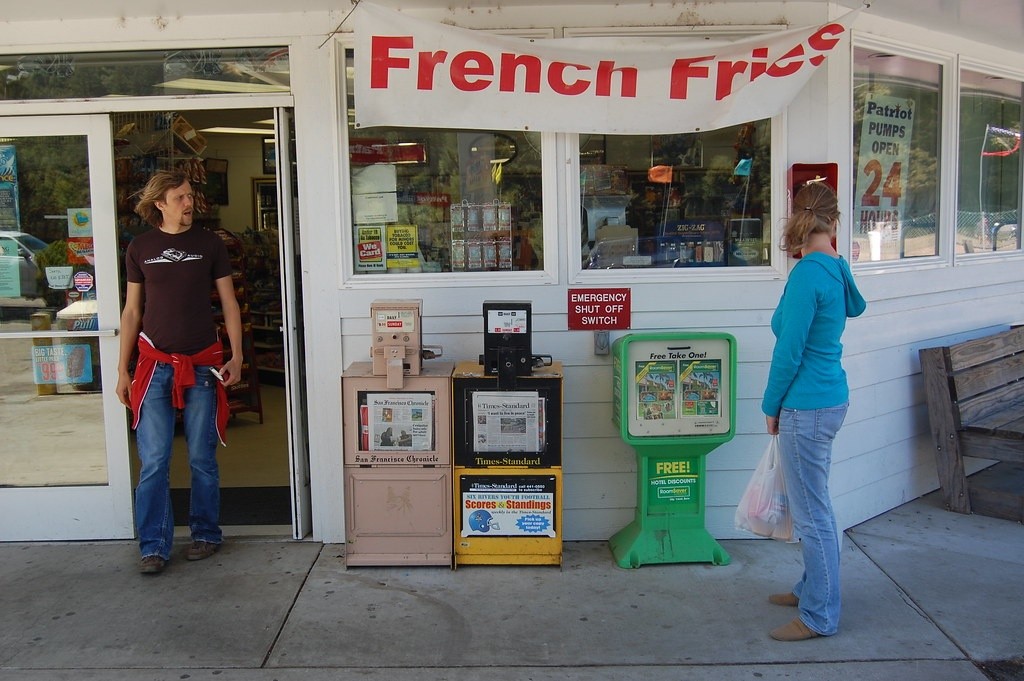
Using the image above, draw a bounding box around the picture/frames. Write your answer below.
[261,137,275,175]
[468,134,518,169]
[650,135,703,170]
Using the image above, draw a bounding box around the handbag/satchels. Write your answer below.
[733,435,793,540]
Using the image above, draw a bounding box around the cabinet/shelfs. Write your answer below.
[680,171,732,220]
[580,194,634,242]
[109,111,286,431]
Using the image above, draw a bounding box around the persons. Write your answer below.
[762,181,865,642]
[116,167,244,574]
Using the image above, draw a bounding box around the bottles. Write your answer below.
[661,241,722,264]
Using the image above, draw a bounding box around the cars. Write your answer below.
[0,230,68,311]
[630,192,764,233]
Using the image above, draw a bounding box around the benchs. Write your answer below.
[920,323,1024,524]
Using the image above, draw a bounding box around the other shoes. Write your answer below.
[185,541,219,561]
[139,556,165,573]
[768,592,799,606]
[769,615,818,642]
